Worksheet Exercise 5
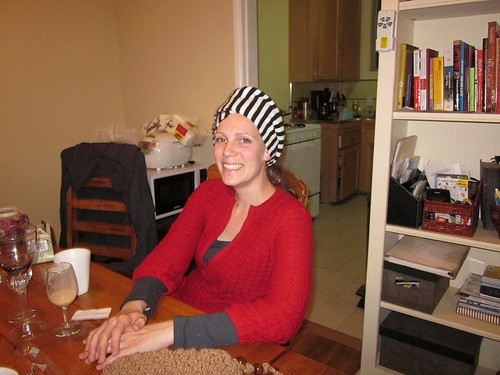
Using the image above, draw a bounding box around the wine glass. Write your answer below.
[0,266,38,323]
[45,262,81,337]
[0,224,49,341]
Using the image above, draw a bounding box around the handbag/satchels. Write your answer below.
[103,348,283,375]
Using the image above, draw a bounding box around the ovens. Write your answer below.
[276,126,321,220]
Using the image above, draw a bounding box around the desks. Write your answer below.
[0,245,343,375]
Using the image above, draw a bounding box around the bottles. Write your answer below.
[320,92,349,119]
[294,97,319,120]
[0,208,26,235]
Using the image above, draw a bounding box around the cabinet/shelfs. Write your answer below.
[357,0,500,375]
[258,0,361,83]
[358,116,375,193]
[292,119,360,204]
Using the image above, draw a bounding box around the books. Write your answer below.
[385,235,470,279]
[399,155,421,183]
[436,174,470,226]
[391,135,417,179]
[455,266,500,326]
[395,20,500,114]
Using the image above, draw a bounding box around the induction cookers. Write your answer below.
[278,110,320,131]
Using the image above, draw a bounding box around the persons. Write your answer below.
[78,86,312,369]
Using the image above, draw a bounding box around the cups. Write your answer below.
[53,248,90,296]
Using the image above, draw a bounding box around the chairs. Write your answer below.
[61,143,143,277]
[207,162,309,211]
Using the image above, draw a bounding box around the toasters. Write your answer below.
[137,129,192,172]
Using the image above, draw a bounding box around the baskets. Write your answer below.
[421,181,482,237]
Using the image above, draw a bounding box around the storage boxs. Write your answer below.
[386,164,431,229]
[422,180,483,238]
[378,311,483,375]
[382,262,450,314]
